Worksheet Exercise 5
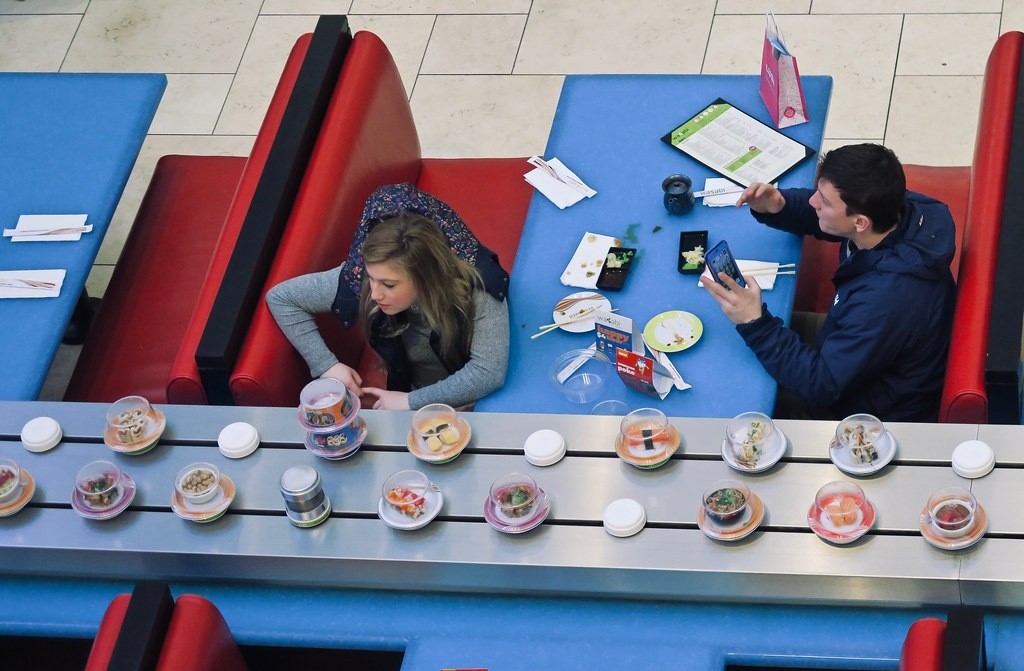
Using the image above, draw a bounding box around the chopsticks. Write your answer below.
[740,263,795,277]
[530,303,619,339]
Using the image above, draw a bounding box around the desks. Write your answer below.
[468,65,834,424]
[0,65,177,405]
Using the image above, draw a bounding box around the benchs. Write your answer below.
[825,27,1024,424]
[898,602,992,671]
[63,15,541,417]
[86,567,147,671]
[143,571,236,671]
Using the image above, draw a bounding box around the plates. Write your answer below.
[171,475,236,520]
[643,311,703,353]
[552,292,611,333]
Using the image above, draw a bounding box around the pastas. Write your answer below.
[706,488,745,517]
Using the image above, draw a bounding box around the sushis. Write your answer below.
[118,409,146,442]
[420,420,459,452]
[626,428,669,449]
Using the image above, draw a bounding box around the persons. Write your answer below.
[697,146,958,423]
[265,213,511,410]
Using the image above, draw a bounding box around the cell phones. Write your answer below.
[705,239,746,292]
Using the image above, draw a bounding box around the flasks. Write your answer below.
[279,464,328,523]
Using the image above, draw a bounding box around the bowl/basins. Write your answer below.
[71,460,136,521]
[297,377,367,461]
[405,404,471,464]
[378,469,443,531]
[483,472,550,534]
[919,487,988,550]
[548,348,612,403]
[0,458,36,517]
[174,461,220,503]
[807,481,875,544]
[103,396,166,455]
[615,408,681,470]
[720,413,788,473]
[828,413,896,476]
[697,479,764,542]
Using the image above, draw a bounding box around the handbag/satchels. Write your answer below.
[758,10,810,130]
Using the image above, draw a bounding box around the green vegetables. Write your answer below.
[748,422,762,461]
[90,478,119,502]
[708,490,736,513]
[616,251,633,264]
[507,490,530,505]
[681,263,698,269]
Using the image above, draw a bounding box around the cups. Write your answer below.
[590,399,631,416]
